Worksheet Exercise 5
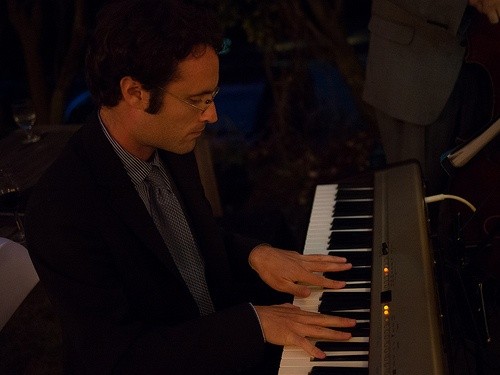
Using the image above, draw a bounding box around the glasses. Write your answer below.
[153,84,220,115]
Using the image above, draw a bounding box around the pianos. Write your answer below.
[278,156,447,375]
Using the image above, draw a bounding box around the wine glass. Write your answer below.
[12,98,41,143]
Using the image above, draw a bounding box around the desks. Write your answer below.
[0,124,84,196]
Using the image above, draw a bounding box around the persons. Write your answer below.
[25,0,357,375]
[365,0,500,167]
